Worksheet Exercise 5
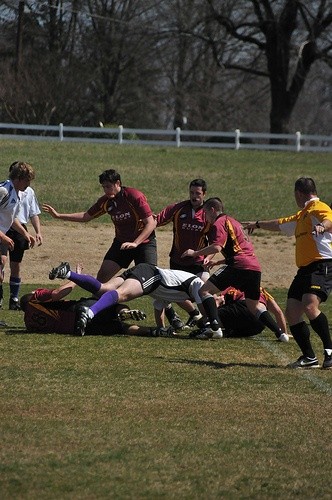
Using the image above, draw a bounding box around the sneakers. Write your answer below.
[9,297,22,311]
[322,350,332,369]
[147,311,289,342]
[75,305,89,336]
[291,356,318,368]
[118,310,146,321]
[48,262,70,279]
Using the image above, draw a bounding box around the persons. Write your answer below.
[48,263,220,337]
[1,162,45,313]
[242,177,332,370]
[191,285,295,343]
[42,170,185,330]
[19,263,180,338]
[182,197,291,344]
[2,163,38,329]
[149,180,218,330]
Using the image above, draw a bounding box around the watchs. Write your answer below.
[316,223,326,234]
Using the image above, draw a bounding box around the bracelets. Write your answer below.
[256,220,262,230]
